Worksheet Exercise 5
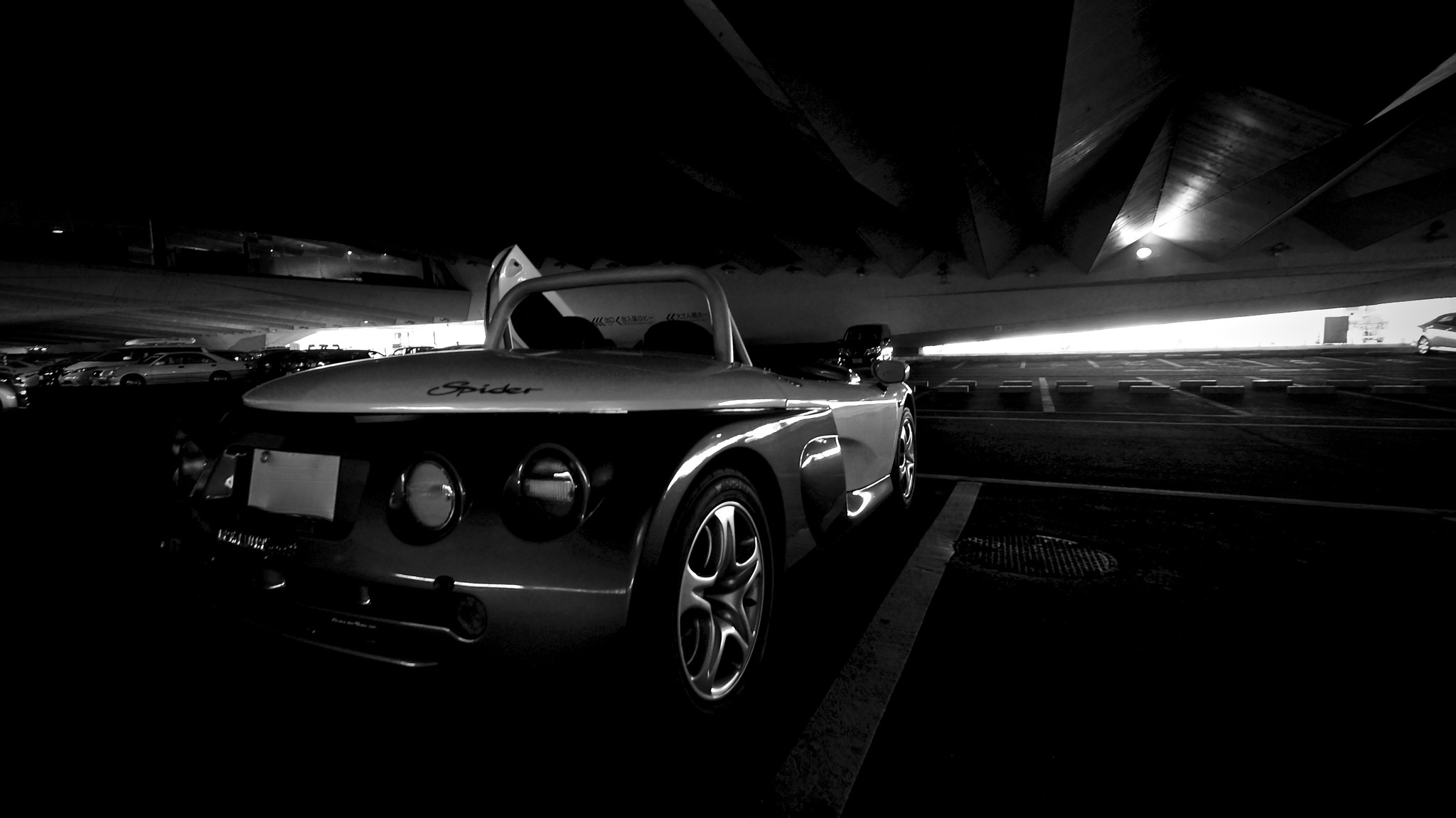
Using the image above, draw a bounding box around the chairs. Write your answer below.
[519,315,606,349]
[640,320,716,358]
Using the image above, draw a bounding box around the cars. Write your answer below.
[163,268,920,718]
[0,346,477,419]
[1408,312,1456,355]
